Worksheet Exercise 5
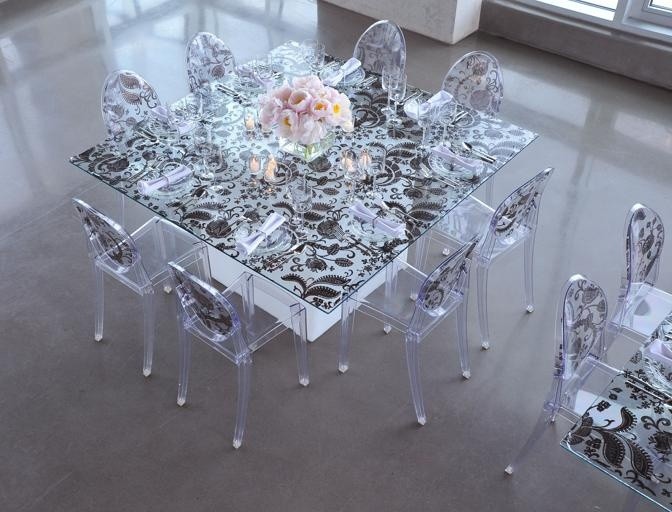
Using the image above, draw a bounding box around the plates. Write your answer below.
[136,169,194,200]
[233,221,291,255]
[403,97,422,122]
[426,145,489,180]
[147,106,203,141]
[319,61,365,86]
[348,201,406,241]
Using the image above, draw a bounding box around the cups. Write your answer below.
[187,93,205,121]
[301,41,324,75]
[248,153,279,182]
[243,113,274,134]
[235,55,255,86]
[255,55,274,79]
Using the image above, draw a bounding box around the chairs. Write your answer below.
[505,273,671,511]
[594,201,671,368]
[69,20,553,449]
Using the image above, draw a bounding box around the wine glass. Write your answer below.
[285,165,312,239]
[204,143,225,197]
[381,64,400,116]
[340,147,385,205]
[415,101,461,153]
[389,73,406,125]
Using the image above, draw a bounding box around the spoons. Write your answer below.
[462,140,496,161]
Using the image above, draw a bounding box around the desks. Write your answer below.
[560,307,669,510]
